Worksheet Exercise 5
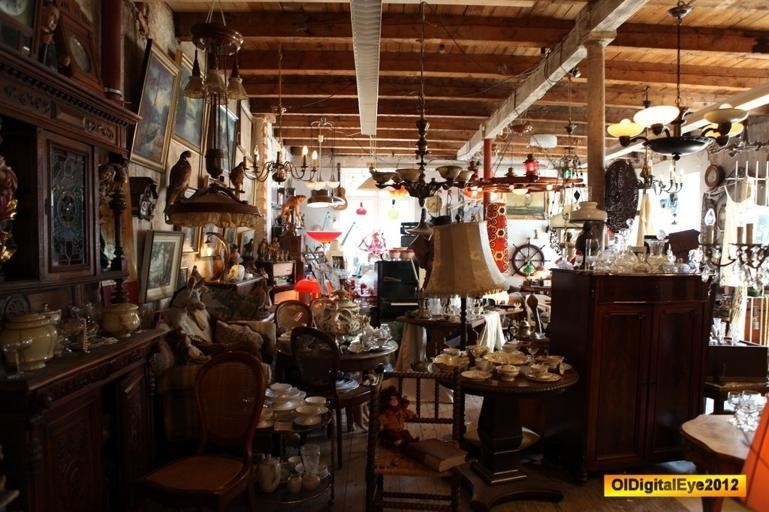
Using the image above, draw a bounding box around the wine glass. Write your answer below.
[728,389,766,431]
[3,336,36,380]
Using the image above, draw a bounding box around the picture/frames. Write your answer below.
[129,38,257,305]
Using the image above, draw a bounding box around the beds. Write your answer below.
[677,411,769,512]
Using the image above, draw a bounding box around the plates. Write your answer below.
[460,354,571,382]
[498,350,520,354]
[296,462,324,475]
[258,388,330,431]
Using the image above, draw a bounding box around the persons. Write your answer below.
[38,4,60,65]
[373,386,419,438]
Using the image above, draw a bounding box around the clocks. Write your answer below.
[59,13,105,93]
[0,0,39,30]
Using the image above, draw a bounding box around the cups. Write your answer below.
[449,297,461,308]
[300,442,320,481]
[429,298,440,315]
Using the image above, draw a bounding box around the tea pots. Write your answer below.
[251,453,282,492]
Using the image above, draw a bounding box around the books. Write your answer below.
[407,438,469,472]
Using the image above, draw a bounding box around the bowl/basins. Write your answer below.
[433,357,470,372]
[548,355,565,364]
[502,345,516,352]
[443,349,460,357]
[531,364,549,377]
[269,382,292,394]
[475,358,488,369]
[495,365,520,381]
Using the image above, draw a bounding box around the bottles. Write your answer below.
[585,239,673,273]
[557,248,574,271]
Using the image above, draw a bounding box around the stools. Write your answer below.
[697,380,769,416]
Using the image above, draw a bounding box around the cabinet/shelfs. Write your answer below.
[537,266,716,487]
[272,233,307,284]
[0,43,146,286]
[0,326,171,512]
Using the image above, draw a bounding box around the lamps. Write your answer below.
[305,230,343,292]
[422,219,511,456]
[179,2,250,104]
[240,41,337,186]
[305,113,347,210]
